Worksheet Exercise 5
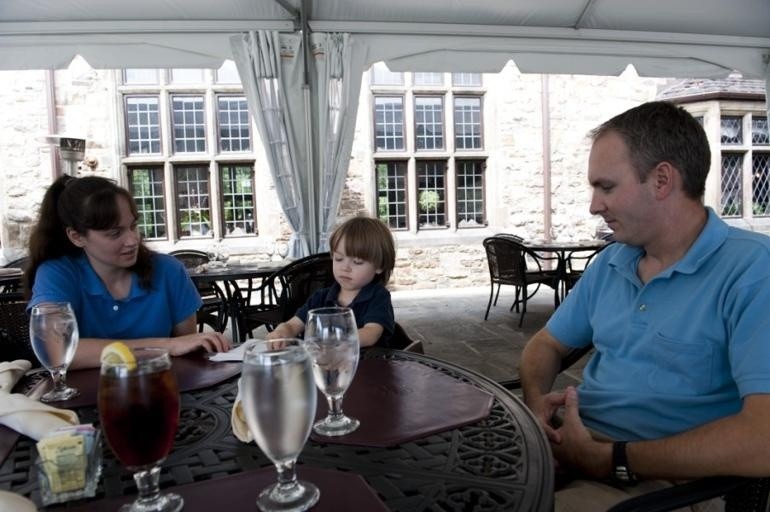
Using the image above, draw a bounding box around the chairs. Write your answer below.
[389,321,422,351]
[0,248,334,370]
[495,339,770,512]
[483,232,614,322]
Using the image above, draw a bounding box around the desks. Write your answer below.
[0,343,556,511]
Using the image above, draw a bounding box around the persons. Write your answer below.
[20,173,236,372]
[263,216,396,355]
[504,95,770,511]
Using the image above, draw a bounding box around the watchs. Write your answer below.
[610,440,634,484]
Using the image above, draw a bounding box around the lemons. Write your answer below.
[100,342,137,370]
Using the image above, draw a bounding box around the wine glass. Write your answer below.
[219,245,231,271]
[97,347,185,512]
[265,242,277,265]
[240,338,321,512]
[278,241,290,265]
[304,306,360,437]
[207,245,217,269]
[29,301,80,402]
